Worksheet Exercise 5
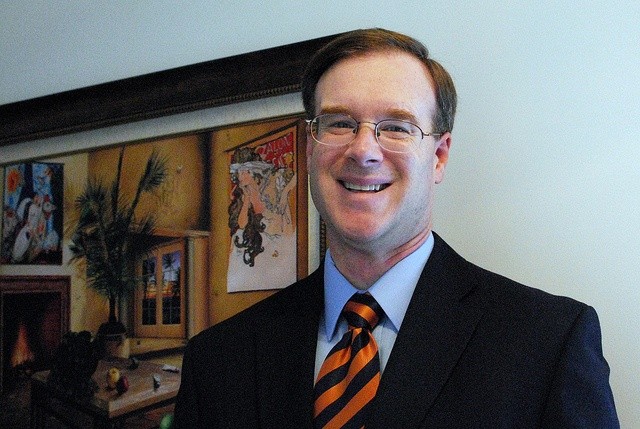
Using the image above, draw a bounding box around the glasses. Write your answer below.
[302,113,441,155]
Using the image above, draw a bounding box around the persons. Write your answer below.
[175,30,622,427]
[231,147,297,237]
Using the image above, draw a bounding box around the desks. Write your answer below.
[25,356,182,429]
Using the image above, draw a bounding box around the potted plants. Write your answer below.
[67,144,167,360]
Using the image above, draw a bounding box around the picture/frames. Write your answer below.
[0,29,362,429]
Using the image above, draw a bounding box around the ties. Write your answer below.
[310,292,381,428]
